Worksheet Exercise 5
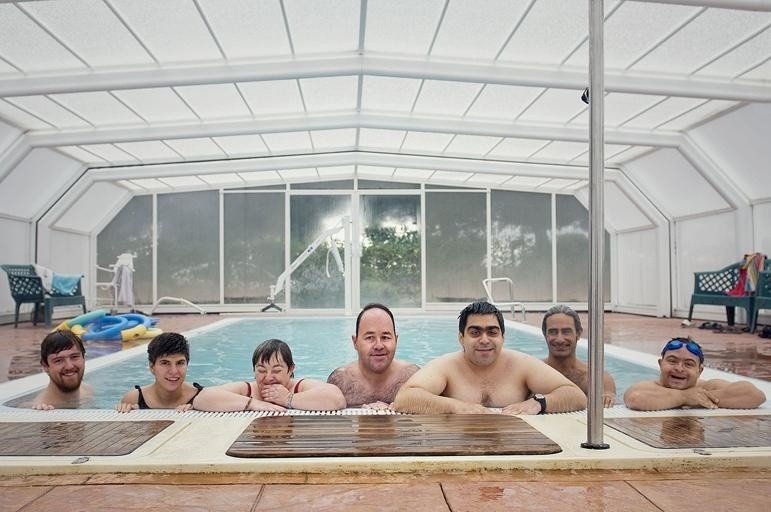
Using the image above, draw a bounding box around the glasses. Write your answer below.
[661,340,701,356]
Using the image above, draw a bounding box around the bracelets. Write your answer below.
[243,396,253,412]
[287,393,293,409]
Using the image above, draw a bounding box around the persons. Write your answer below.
[538,303,617,409]
[392,301,588,415]
[326,304,421,411]
[192,338,347,412]
[623,337,761,411]
[114,332,204,414]
[17,330,95,411]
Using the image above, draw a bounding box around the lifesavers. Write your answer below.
[51,309,162,342]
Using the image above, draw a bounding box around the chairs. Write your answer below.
[748,259,771,336]
[689,257,766,330]
[1,264,86,327]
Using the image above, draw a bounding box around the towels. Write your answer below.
[53,273,85,297]
[117,253,137,307]
[723,251,768,300]
[32,264,53,295]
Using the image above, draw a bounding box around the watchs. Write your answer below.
[530,393,547,414]
